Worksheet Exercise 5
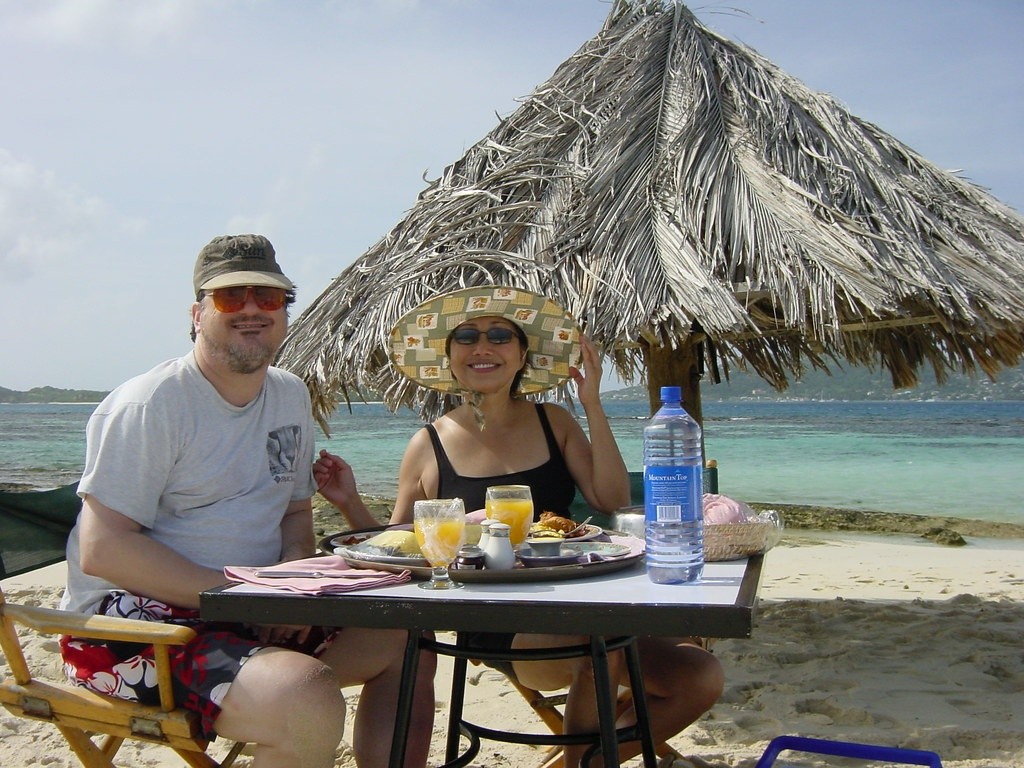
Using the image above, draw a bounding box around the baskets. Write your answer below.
[615,504,775,563]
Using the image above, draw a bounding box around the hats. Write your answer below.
[387,286,583,396]
[194,234,292,294]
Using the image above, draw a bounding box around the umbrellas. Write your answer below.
[268,1,1023,502]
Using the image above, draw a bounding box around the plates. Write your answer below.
[330,531,384,547]
[525,523,603,543]
[515,547,584,568]
[333,547,426,566]
[566,542,632,557]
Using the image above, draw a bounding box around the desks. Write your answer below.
[200,519,764,768]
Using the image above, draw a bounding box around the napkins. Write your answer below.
[224,555,412,594]
[465,510,488,525]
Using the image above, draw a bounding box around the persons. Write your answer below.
[313,284,725,768]
[57,235,438,768]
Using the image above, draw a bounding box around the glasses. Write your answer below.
[204,285,285,313]
[451,328,521,345]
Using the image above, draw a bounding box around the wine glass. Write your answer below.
[485,485,534,566]
[413,499,466,590]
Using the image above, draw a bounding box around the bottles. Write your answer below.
[644,387,705,585]
[477,519,500,548]
[484,523,513,569]
[455,545,483,570]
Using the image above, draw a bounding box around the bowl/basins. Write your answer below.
[524,537,565,556]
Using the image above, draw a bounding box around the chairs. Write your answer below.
[464,458,720,768]
[0,478,247,768]
